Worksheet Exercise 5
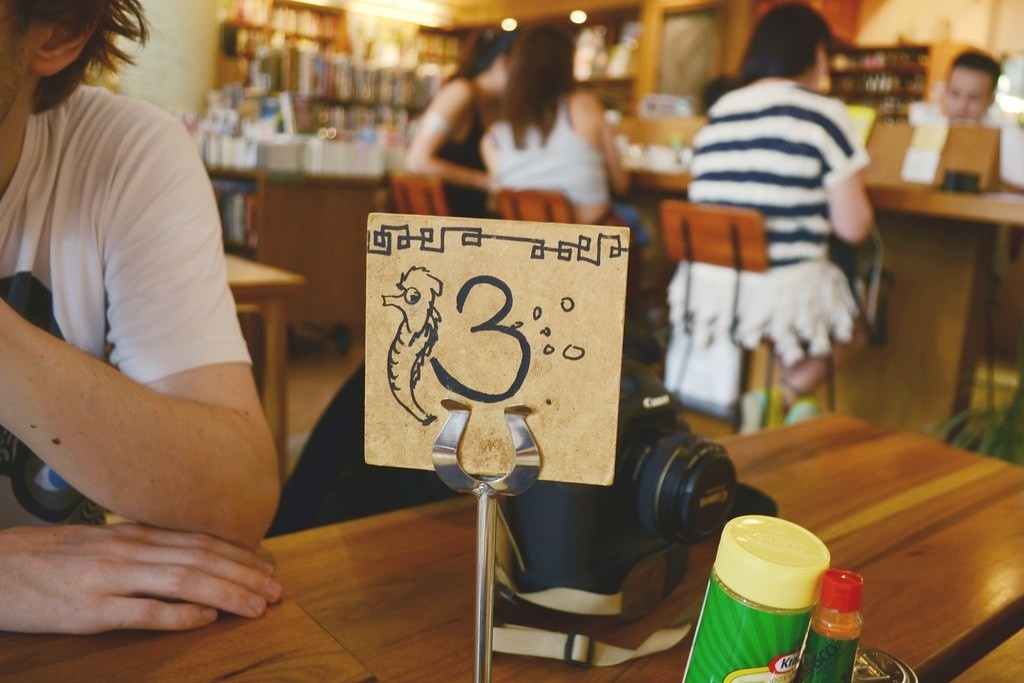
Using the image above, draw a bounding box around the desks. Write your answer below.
[224,256,307,483]
[0,409,1024,683]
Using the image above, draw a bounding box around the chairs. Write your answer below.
[389,171,451,219]
[495,190,573,222]
[661,200,837,433]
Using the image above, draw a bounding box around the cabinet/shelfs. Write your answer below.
[214,0,461,138]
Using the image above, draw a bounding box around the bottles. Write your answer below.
[681,512,865,683]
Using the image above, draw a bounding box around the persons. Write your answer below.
[932,52,1024,191]
[687,4,873,432]
[478,22,654,250]
[1,0,284,636]
[408,28,521,220]
